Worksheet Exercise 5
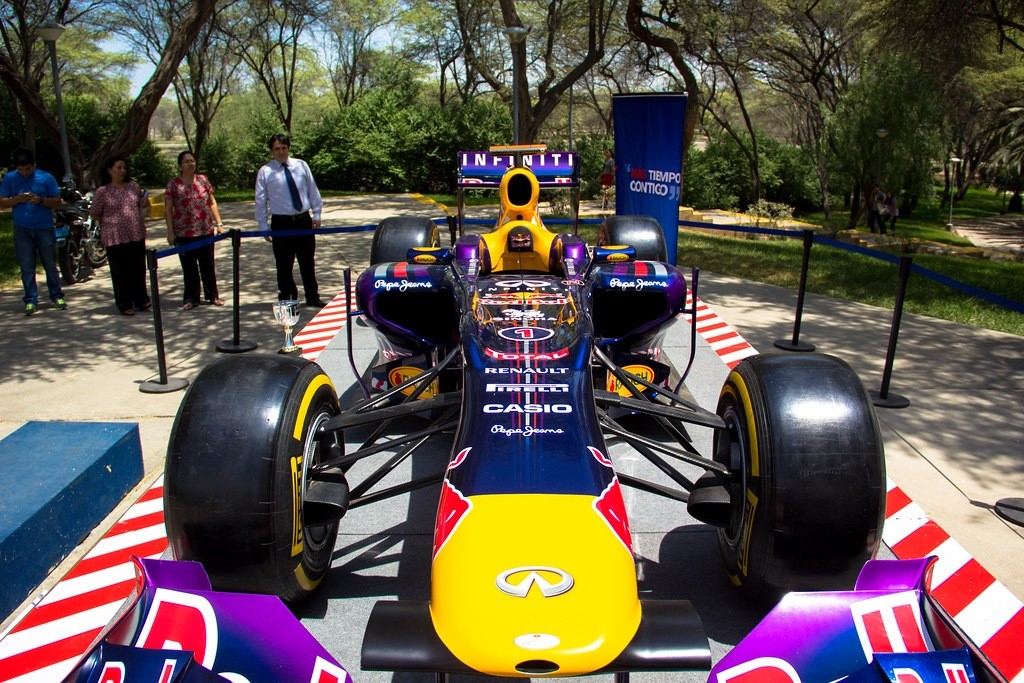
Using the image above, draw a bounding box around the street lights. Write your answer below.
[875,128,890,185]
[501,27,530,145]
[562,65,574,152]
[946,155,962,230]
[35,19,75,183]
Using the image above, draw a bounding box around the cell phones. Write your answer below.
[24,193,30,196]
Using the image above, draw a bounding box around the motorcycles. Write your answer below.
[53,173,108,284]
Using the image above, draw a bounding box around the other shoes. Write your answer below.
[183,301,194,310]
[210,298,223,306]
[24,303,37,314]
[280,301,299,316]
[140,303,151,310]
[54,297,68,310]
[306,297,327,308]
[121,309,135,316]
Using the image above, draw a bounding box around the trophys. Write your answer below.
[273,300,302,357]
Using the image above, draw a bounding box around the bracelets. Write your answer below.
[217,223,222,226]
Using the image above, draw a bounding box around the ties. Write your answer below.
[281,163,303,212]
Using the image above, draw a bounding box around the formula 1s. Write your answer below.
[157,137,889,683]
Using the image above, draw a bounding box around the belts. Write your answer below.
[272,211,310,221]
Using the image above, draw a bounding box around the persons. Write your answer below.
[88,156,152,315]
[597,149,616,210]
[255,133,328,308]
[164,151,225,310]
[869,183,899,238]
[0,156,68,315]
